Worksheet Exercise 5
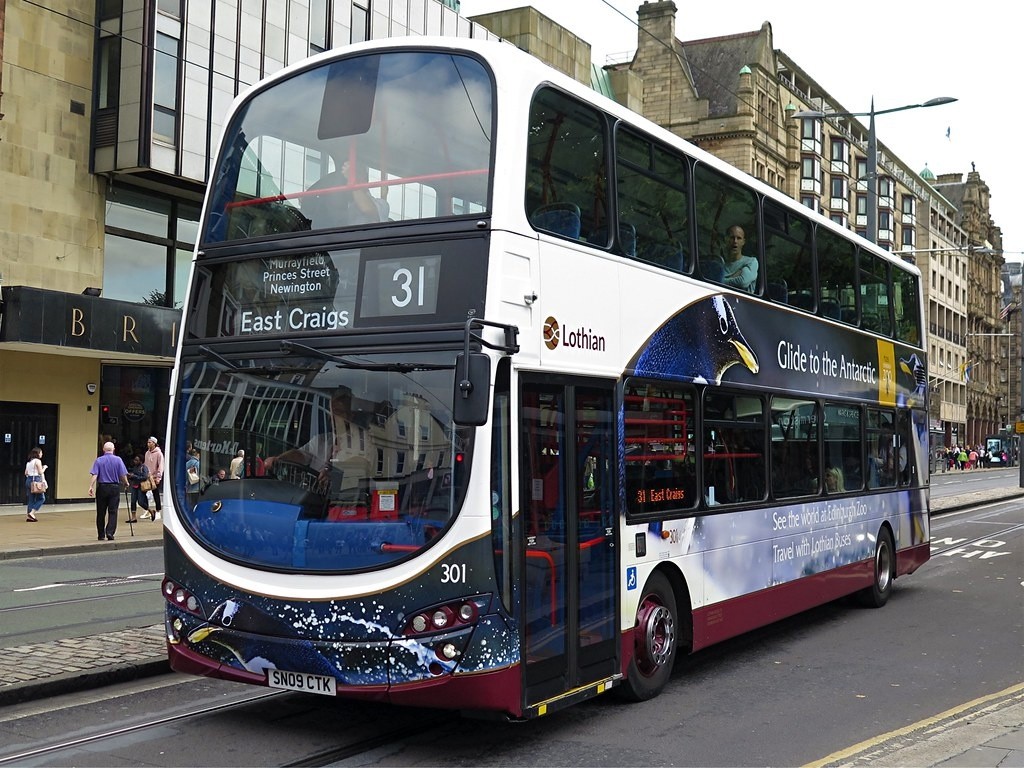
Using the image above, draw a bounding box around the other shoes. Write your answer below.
[125,519,137,523]
[152,510,156,521]
[26,513,38,522]
[107,533,114,540]
[98,536,105,540]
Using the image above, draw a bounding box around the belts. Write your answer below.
[98,484,119,487]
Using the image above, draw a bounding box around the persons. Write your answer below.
[942,442,1012,471]
[212,470,227,481]
[824,465,845,493]
[230,441,264,480]
[186,440,199,493]
[88,442,129,540]
[25,447,49,522]
[140,436,165,520]
[724,226,759,293]
[298,160,390,231]
[125,454,158,523]
[265,394,377,504]
[877,425,908,487]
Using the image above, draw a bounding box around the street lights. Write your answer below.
[788,95,958,325]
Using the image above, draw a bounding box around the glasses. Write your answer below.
[825,463,840,476]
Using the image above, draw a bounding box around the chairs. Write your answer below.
[531,202,883,337]
[623,449,911,514]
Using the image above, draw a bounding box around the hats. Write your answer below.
[148,436,159,446]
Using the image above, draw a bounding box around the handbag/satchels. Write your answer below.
[31,481,46,494]
[140,473,157,492]
[955,451,960,458]
[186,466,200,485]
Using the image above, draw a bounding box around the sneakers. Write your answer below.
[139,511,152,519]
[154,512,161,520]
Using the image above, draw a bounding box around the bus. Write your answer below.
[157,33,938,725]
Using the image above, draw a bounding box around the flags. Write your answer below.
[960,363,975,381]
